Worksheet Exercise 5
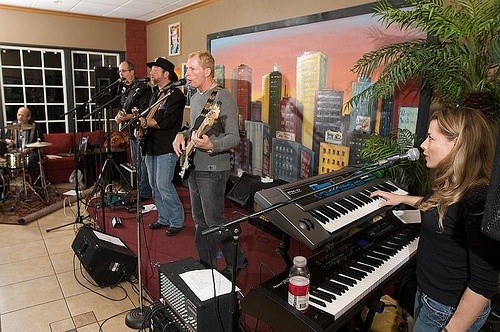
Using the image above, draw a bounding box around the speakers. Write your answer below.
[227,172,290,240]
[71,227,137,287]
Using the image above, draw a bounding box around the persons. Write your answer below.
[370,105,498,332]
[172,50,248,279]
[6,107,44,196]
[115,61,152,201]
[134,57,185,236]
[171,27,179,54]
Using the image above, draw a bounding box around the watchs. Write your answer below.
[142,118,147,129]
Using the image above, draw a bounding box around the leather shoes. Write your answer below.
[166,226,185,235]
[148,221,164,229]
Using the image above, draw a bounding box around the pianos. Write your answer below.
[241,207,424,332]
[253,162,411,250]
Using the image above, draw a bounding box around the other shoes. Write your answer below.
[220,255,249,278]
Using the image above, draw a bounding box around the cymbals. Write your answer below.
[25,141,54,148]
[3,123,34,130]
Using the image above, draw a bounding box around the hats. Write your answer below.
[145,57,178,82]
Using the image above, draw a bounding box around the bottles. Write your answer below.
[288,256,310,314]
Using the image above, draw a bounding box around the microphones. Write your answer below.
[106,78,123,89]
[162,79,186,92]
[131,77,151,82]
[379,148,420,167]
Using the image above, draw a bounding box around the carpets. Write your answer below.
[0,184,93,225]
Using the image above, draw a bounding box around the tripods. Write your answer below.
[10,83,141,232]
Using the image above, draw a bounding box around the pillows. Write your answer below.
[104,131,129,149]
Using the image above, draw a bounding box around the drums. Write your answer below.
[0,169,10,203]
[0,157,7,168]
[4,152,25,169]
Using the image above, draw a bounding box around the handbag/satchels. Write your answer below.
[361,294,414,332]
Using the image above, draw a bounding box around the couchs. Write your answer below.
[43,130,132,185]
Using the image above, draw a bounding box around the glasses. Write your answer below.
[116,69,132,74]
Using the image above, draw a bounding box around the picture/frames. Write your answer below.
[206,0,436,183]
[181,62,199,110]
[168,22,182,57]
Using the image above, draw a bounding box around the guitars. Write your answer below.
[177,104,221,180]
